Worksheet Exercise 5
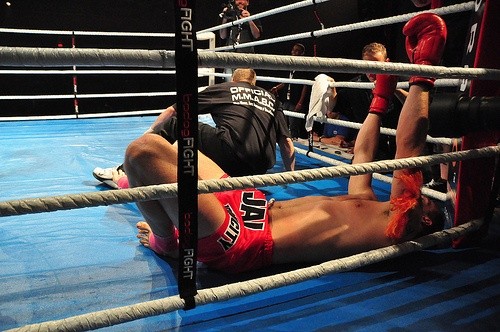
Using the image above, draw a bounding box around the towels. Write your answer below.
[304,74,337,132]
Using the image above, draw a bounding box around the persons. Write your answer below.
[92,0,450,275]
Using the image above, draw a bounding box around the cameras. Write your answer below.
[425,93,500,138]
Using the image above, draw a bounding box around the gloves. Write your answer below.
[402,13,447,86]
[367,72,398,116]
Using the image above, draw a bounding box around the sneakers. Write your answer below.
[93,163,123,189]
